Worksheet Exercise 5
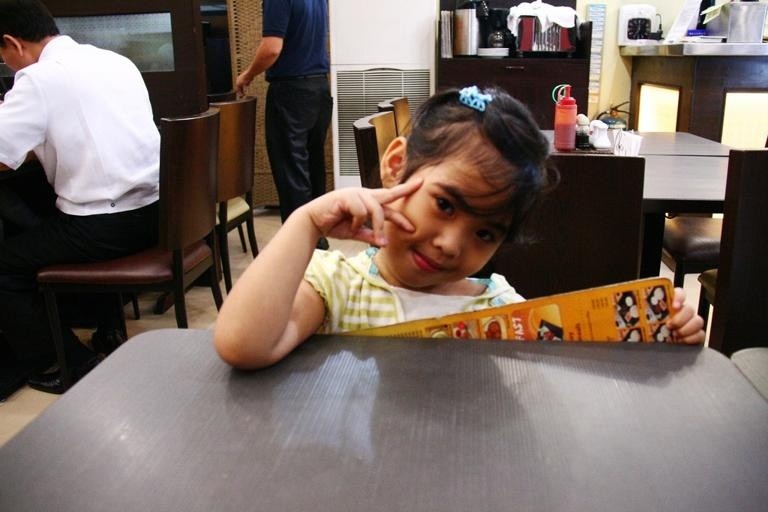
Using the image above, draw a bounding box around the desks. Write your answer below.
[543,130,735,278]
[0,329,768,512]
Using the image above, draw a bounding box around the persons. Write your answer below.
[0,0,160,395]
[233,0,330,250]
[212,86,706,371]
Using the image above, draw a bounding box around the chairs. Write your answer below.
[664,215,723,288]
[354,111,397,189]
[505,157,645,304]
[36,107,223,392]
[210,96,258,295]
[696,150,768,356]
[377,97,410,138]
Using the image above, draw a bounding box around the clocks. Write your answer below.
[618,3,656,46]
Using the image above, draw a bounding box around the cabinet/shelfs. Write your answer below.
[619,56,767,150]
[435,0,592,129]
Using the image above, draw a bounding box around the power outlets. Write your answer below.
[656,12,664,28]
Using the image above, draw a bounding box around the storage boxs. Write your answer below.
[708,2,768,43]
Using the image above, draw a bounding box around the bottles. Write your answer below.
[553,85,577,151]
